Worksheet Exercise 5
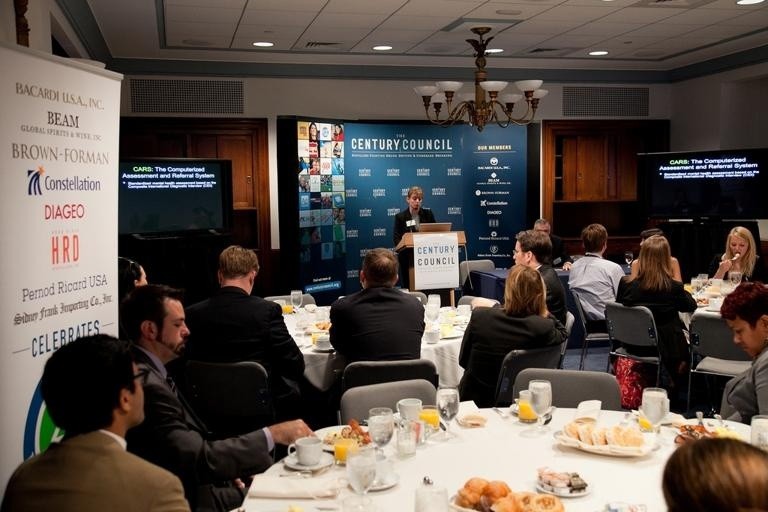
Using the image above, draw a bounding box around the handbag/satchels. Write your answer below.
[612,352,644,412]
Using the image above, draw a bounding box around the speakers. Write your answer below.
[233,209,260,252]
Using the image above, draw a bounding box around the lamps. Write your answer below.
[411,26,551,136]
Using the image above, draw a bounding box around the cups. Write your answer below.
[419,405,440,436]
[681,271,743,312]
[395,419,417,458]
[398,398,423,419]
[517,389,538,425]
[288,437,322,465]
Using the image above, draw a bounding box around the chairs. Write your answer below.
[184,256,767,511]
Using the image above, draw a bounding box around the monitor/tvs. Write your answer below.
[118,156,233,240]
[636,148,768,225]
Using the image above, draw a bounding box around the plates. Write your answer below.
[552,428,666,458]
[536,477,594,498]
[284,452,334,472]
[314,423,372,452]
[272,289,336,355]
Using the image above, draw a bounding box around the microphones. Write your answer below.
[405,213,416,232]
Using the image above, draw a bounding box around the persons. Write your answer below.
[458,218,572,408]
[329,248,426,367]
[615,235,698,395]
[629,228,683,283]
[394,186,436,247]
[719,282,768,425]
[298,122,346,243]
[0,333,190,512]
[567,223,626,333]
[708,226,767,284]
[662,437,768,511]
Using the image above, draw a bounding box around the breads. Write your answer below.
[456,477,564,512]
[316,320,332,330]
[563,422,646,447]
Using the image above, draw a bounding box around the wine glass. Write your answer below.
[346,447,377,512]
[368,407,395,456]
[528,379,553,434]
[415,292,473,345]
[434,384,462,437]
[641,388,668,431]
[625,252,634,269]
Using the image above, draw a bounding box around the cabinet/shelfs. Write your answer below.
[562,136,638,200]
[553,233,642,262]
[187,130,254,209]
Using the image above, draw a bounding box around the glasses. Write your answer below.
[132,366,152,386]
[513,248,524,256]
